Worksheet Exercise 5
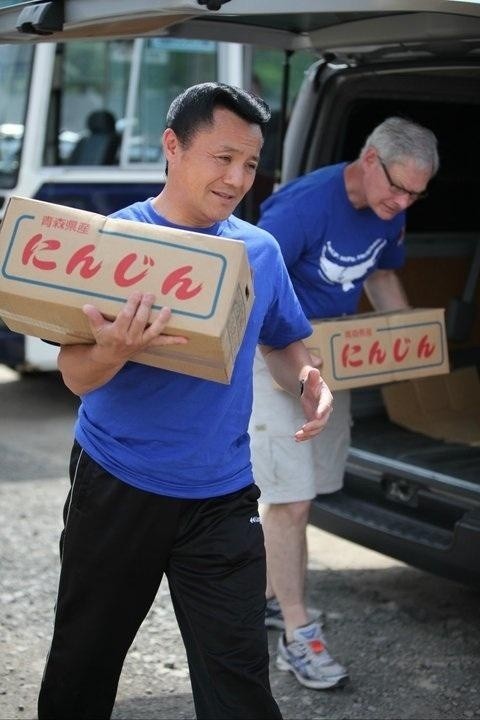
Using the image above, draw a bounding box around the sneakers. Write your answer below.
[262,594,327,631]
[276,623,350,690]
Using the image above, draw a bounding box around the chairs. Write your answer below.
[64,108,122,165]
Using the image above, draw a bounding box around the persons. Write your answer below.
[85,109,123,148]
[33,80,338,720]
[252,114,443,692]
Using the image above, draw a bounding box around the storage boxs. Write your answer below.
[299,302,452,394]
[2,195,256,387]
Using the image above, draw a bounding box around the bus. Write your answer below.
[1,39,318,375]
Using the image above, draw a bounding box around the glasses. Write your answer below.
[375,151,427,201]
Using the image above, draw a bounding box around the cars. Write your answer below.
[1,0,480,581]
